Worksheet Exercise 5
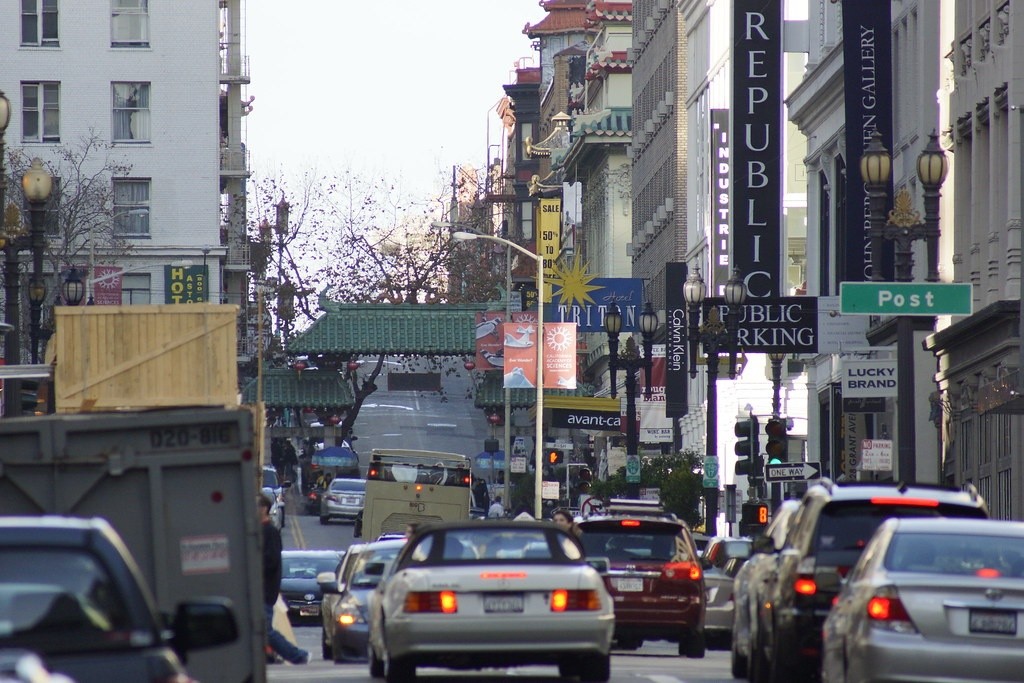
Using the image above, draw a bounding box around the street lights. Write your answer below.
[428,219,513,515]
[602,300,660,502]
[764,351,790,521]
[452,229,546,522]
[857,124,951,485]
[199,244,212,301]
[25,264,87,415]
[0,157,62,422]
[88,206,149,305]
[683,259,748,538]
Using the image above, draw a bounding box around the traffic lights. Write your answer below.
[740,502,768,525]
[577,467,594,494]
[734,414,764,477]
[762,417,789,464]
[545,450,564,465]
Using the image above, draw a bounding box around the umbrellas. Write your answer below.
[311,446,358,477]
[475,450,511,484]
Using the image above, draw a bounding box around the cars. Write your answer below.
[362,518,614,683]
[262,462,292,533]
[278,534,484,666]
[820,518,1024,683]
[320,477,366,523]
[601,534,755,650]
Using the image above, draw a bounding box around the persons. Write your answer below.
[256,493,313,666]
[554,510,576,532]
[315,468,333,489]
[511,504,536,521]
[472,470,490,517]
[497,470,504,484]
[486,494,506,519]
[271,436,298,484]
[543,500,556,518]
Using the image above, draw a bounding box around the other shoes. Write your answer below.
[300,652,313,665]
[274,655,284,665]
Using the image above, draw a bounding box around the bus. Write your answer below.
[359,445,477,542]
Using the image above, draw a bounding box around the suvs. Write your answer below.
[749,474,995,683]
[723,497,800,678]
[0,513,241,683]
[569,507,713,660]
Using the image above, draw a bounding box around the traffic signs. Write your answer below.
[765,462,822,484]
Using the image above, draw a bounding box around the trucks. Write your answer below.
[1,402,279,683]
